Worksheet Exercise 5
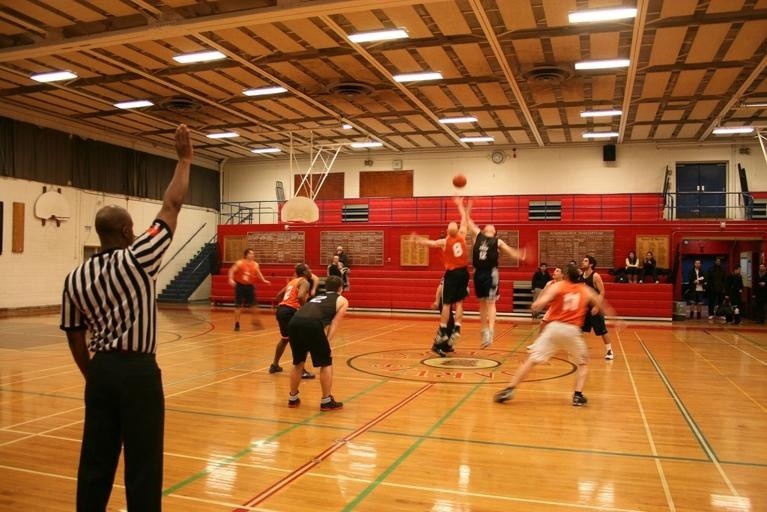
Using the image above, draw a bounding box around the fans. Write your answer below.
[523,66,574,84]
[159,96,205,113]
[326,81,375,97]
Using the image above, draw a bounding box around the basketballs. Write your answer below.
[452,176,467,188]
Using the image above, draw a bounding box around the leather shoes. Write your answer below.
[735,317,742,323]
[689,312,693,318]
[725,317,732,322]
[697,313,702,319]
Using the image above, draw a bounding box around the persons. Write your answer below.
[726,265,743,325]
[327,256,349,293]
[432,277,455,358]
[307,271,319,303]
[493,263,627,406]
[753,264,767,323]
[287,277,348,410]
[579,254,614,361]
[532,263,554,320]
[707,257,726,319]
[334,247,348,267]
[454,196,526,349]
[269,263,315,379]
[640,251,660,284]
[230,249,270,331]
[564,260,582,283]
[626,250,639,283]
[527,267,565,346]
[690,260,707,318]
[59,124,192,511]
[413,197,472,346]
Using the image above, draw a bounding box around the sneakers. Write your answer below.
[526,343,533,350]
[656,280,659,283]
[494,392,513,402]
[639,280,642,283]
[573,395,588,405]
[633,280,637,282]
[490,335,493,344]
[269,364,283,373]
[628,280,630,283]
[709,314,712,317]
[480,334,490,349]
[605,352,614,361]
[433,343,453,358]
[319,398,343,411]
[719,316,724,318]
[234,322,240,330]
[454,328,461,338]
[433,328,449,342]
[301,370,316,379]
[288,398,301,408]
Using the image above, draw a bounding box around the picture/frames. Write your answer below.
[393,159,401,168]
[493,152,503,163]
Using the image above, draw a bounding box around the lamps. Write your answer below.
[281,131,319,223]
